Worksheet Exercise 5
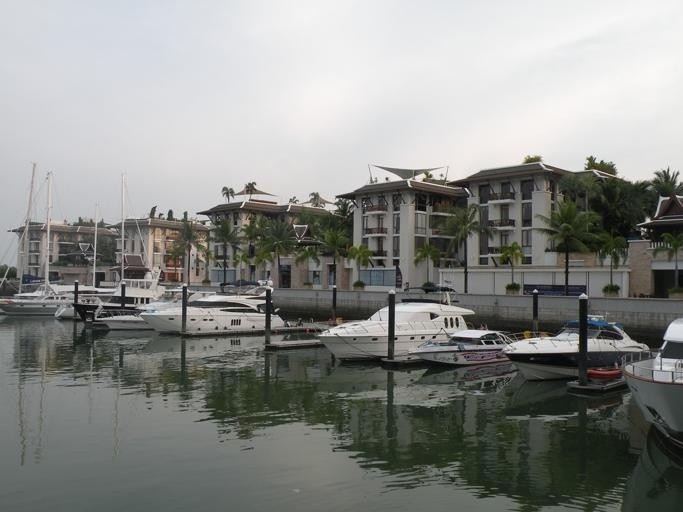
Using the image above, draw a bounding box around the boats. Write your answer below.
[1,315,265,376]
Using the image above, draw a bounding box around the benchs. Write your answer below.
[523,284,586,296]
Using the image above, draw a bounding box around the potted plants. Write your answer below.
[668,287,683,299]
[601,283,621,297]
[353,281,366,290]
[505,282,521,295]
[202,279,211,285]
[304,281,314,289]
